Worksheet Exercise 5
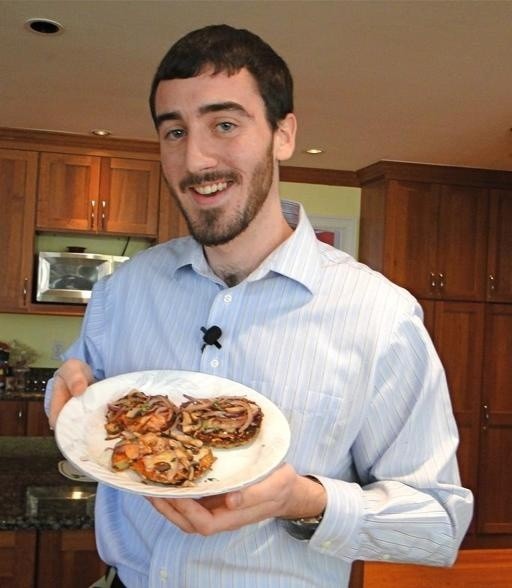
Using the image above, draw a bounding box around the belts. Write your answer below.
[105,566,125,587]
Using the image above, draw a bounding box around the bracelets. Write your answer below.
[278,511,323,542]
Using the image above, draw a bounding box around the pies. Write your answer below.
[108,393,263,485]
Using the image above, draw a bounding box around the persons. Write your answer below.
[42,23,479,586]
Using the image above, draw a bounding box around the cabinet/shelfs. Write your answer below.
[355,160,512,549]
[1,401,27,436]
[0,126,190,317]
[27,403,54,437]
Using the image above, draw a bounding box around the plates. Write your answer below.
[56,369,292,501]
[57,459,100,484]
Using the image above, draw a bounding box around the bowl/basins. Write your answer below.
[25,487,92,518]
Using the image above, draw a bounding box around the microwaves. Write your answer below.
[36,251,134,305]
